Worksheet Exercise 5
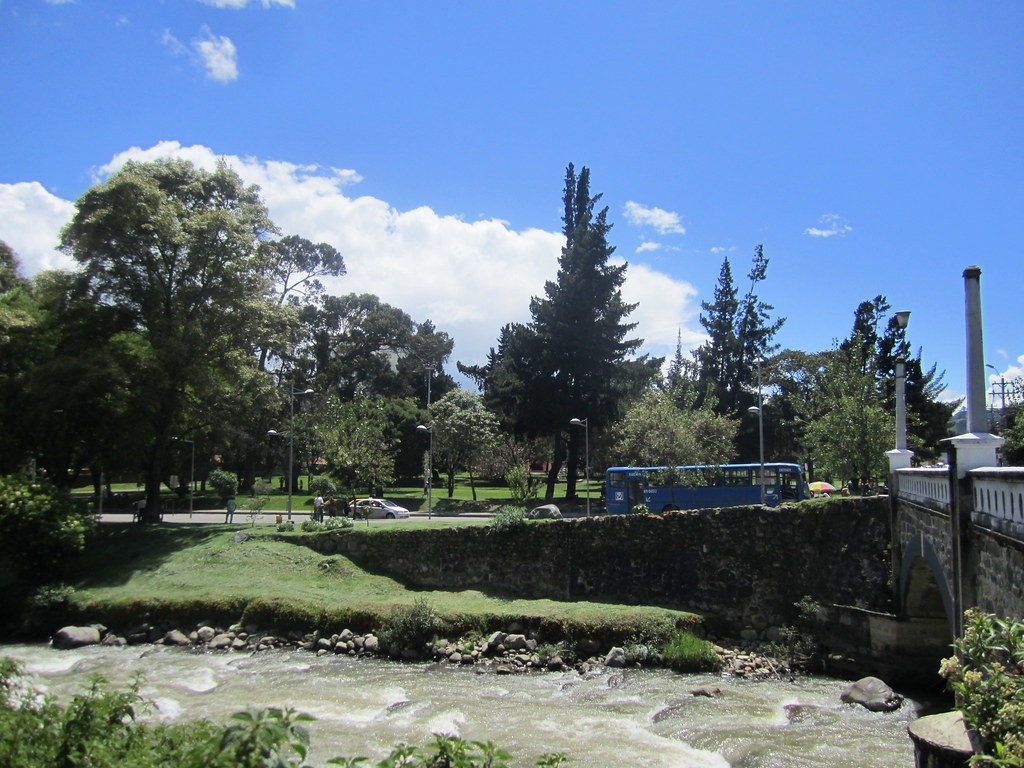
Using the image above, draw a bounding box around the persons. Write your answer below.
[810,491,831,498]
[225,496,237,524]
[314,494,324,523]
[322,495,340,518]
[343,497,352,517]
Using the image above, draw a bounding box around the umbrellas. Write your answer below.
[808,481,836,492]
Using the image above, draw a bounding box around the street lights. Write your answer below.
[172,437,195,518]
[267,381,314,523]
[748,346,765,504]
[986,364,1005,407]
[570,418,590,517]
[895,311,911,450]
[416,364,435,520]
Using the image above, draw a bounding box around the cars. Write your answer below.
[347,499,377,506]
[425,479,443,487]
[78,468,91,477]
[193,491,205,496]
[348,497,410,519]
[321,499,345,514]
[874,486,889,494]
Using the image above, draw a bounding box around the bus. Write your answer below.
[605,463,811,515]
